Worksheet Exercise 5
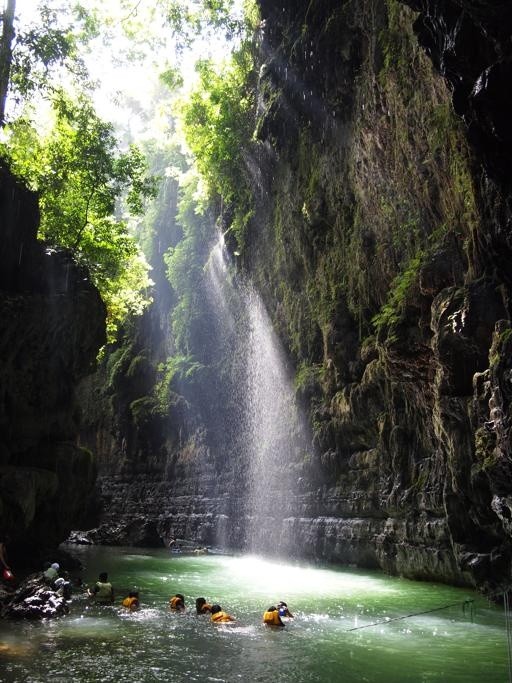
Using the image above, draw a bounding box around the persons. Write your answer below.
[194,546,208,555]
[195,597,212,614]
[264,606,284,625]
[170,543,182,554]
[0,542,16,589]
[86,572,115,604]
[123,590,140,608]
[42,562,82,591]
[276,601,293,617]
[170,593,185,611]
[210,605,235,623]
